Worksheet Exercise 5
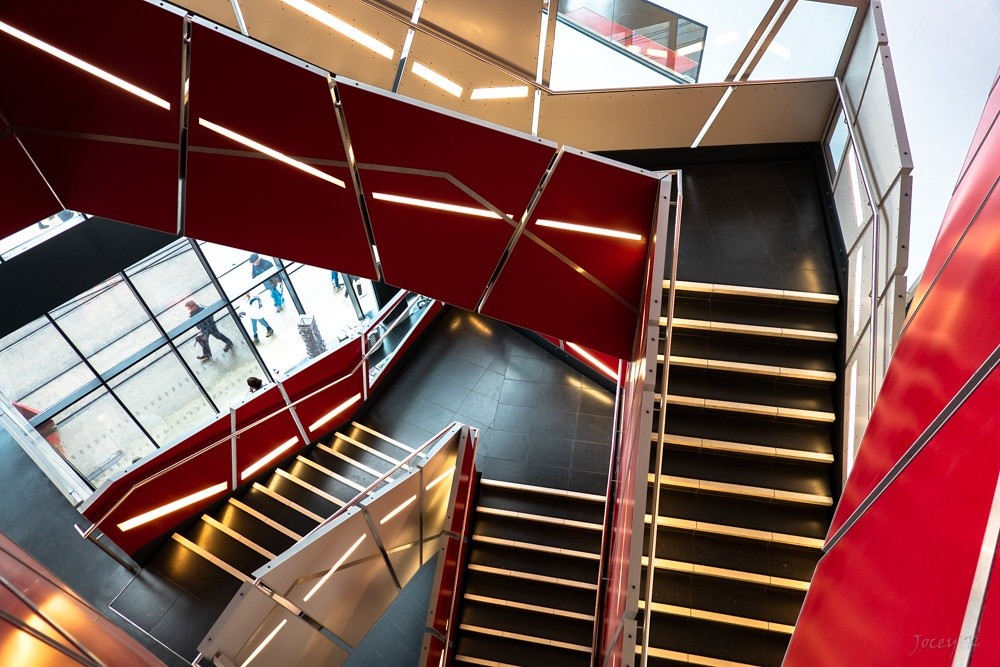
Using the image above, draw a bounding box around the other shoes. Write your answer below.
[196,354,212,361]
[222,342,233,352]
[252,337,260,344]
[265,328,273,337]
[276,305,283,312]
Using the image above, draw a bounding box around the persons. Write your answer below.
[246,377,267,393]
[238,292,273,345]
[250,254,285,313]
[330,270,368,304]
[185,300,233,360]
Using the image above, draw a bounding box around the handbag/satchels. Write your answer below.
[195,335,208,346]
